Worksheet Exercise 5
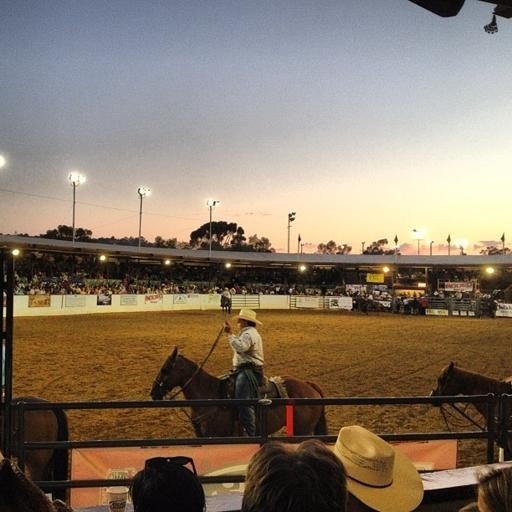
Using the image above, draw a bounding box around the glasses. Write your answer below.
[143,456,197,480]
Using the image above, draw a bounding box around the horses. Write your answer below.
[428,359,512,462]
[0,394,73,512]
[220,295,233,313]
[148,346,329,437]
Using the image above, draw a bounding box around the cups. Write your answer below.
[106,486,129,512]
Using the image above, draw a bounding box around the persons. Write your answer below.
[241,438,348,512]
[128,456,206,512]
[1,250,512,316]
[476,467,512,512]
[222,308,265,436]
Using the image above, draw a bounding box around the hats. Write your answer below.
[232,308,263,326]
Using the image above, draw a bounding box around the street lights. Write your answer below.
[287,211,296,255]
[207,199,220,254]
[68,173,84,246]
[412,229,427,255]
[457,238,469,255]
[138,186,151,249]
